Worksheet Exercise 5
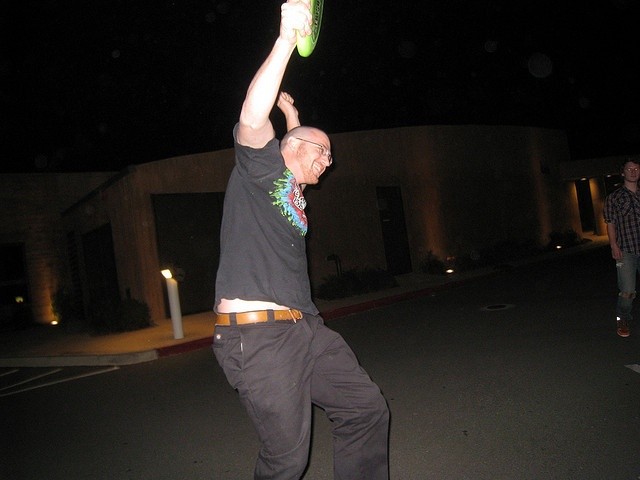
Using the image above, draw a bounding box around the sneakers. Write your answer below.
[616,316,630,337]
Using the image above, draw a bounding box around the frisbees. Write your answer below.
[297,1,324,56]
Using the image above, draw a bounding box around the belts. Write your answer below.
[215,307,305,326]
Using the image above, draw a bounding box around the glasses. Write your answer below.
[623,168,640,172]
[296,138,333,164]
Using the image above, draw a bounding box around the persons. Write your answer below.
[212,0,390,480]
[602,160,640,338]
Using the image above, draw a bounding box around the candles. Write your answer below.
[159,267,186,341]
